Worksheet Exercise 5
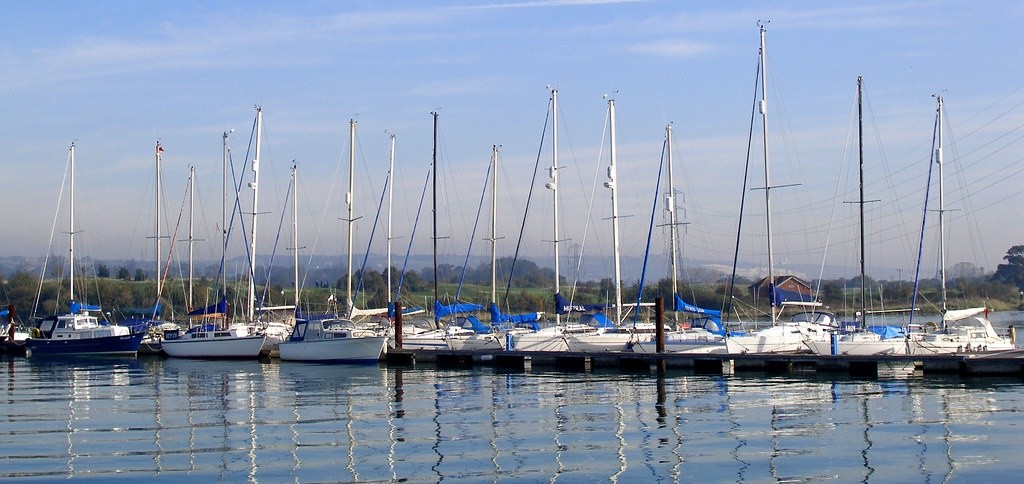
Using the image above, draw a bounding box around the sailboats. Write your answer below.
[24,139,147,358]
[494,83,606,352]
[724,19,841,354]
[800,75,924,355]
[141,104,535,352]
[904,92,1017,355]
[564,90,672,353]
[161,129,267,358]
[623,121,743,353]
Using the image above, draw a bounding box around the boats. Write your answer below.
[276,315,389,364]
